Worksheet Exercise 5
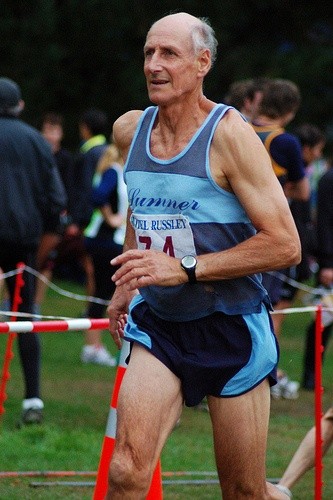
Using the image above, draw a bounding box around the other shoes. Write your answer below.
[265,479,292,500]
[79,344,118,368]
[20,406,46,426]
[270,370,302,401]
[305,385,326,394]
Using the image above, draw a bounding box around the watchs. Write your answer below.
[180,255,198,284]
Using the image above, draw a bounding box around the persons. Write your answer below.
[107,12,302,500]
[1,78,66,426]
[35,78,333,406]
[276,407,333,497]
